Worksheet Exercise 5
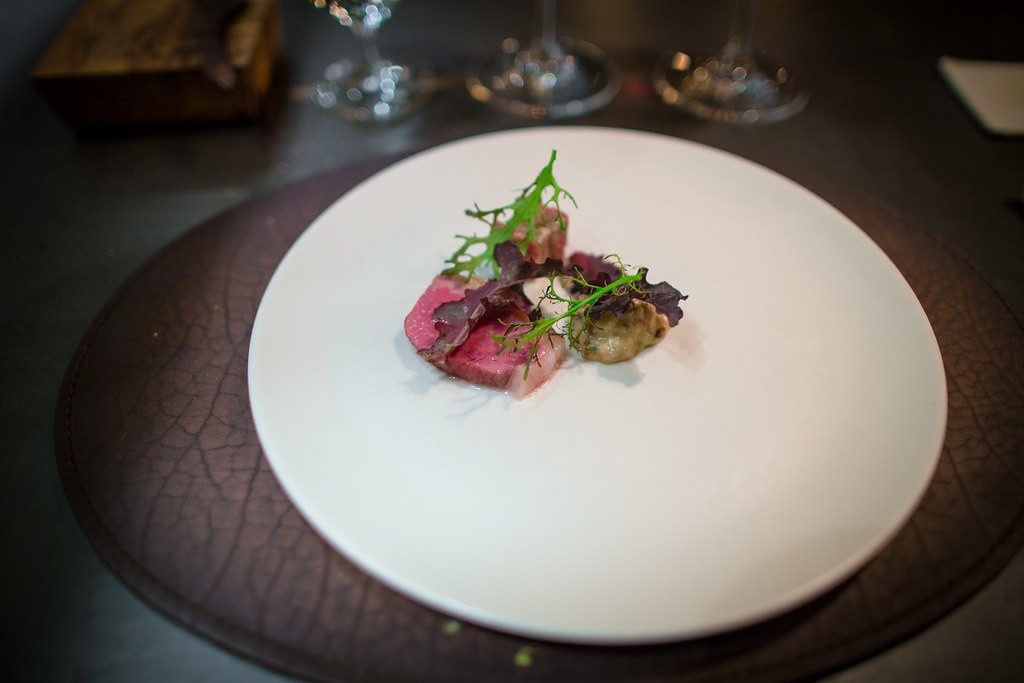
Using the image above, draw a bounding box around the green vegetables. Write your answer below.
[416,149,687,380]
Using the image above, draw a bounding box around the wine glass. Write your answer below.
[463,0,626,120]
[651,0,814,125]
[308,0,434,123]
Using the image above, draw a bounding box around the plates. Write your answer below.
[249,125,949,648]
